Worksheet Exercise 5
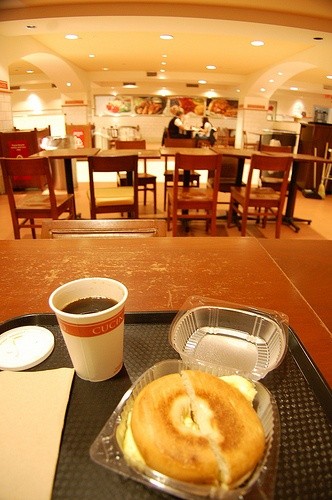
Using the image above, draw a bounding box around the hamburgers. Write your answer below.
[115,371,263,490]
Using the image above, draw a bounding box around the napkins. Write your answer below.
[0,368,75,500]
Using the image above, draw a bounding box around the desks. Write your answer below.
[160,147,217,233]
[96,149,161,186]
[0,238,332,389]
[210,147,271,231]
[262,151,332,233]
[29,148,101,220]
[248,131,273,151]
[181,126,198,139]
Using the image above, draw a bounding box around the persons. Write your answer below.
[195,117,213,140]
[169,111,189,138]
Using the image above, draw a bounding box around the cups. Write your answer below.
[49,277,129,382]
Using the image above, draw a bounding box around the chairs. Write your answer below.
[115,140,157,217]
[166,128,170,137]
[167,152,222,237]
[227,154,293,239]
[88,154,140,220]
[0,156,75,240]
[259,144,293,224]
[243,131,258,150]
[164,137,200,213]
[40,219,168,239]
[106,128,119,149]
[197,126,220,148]
